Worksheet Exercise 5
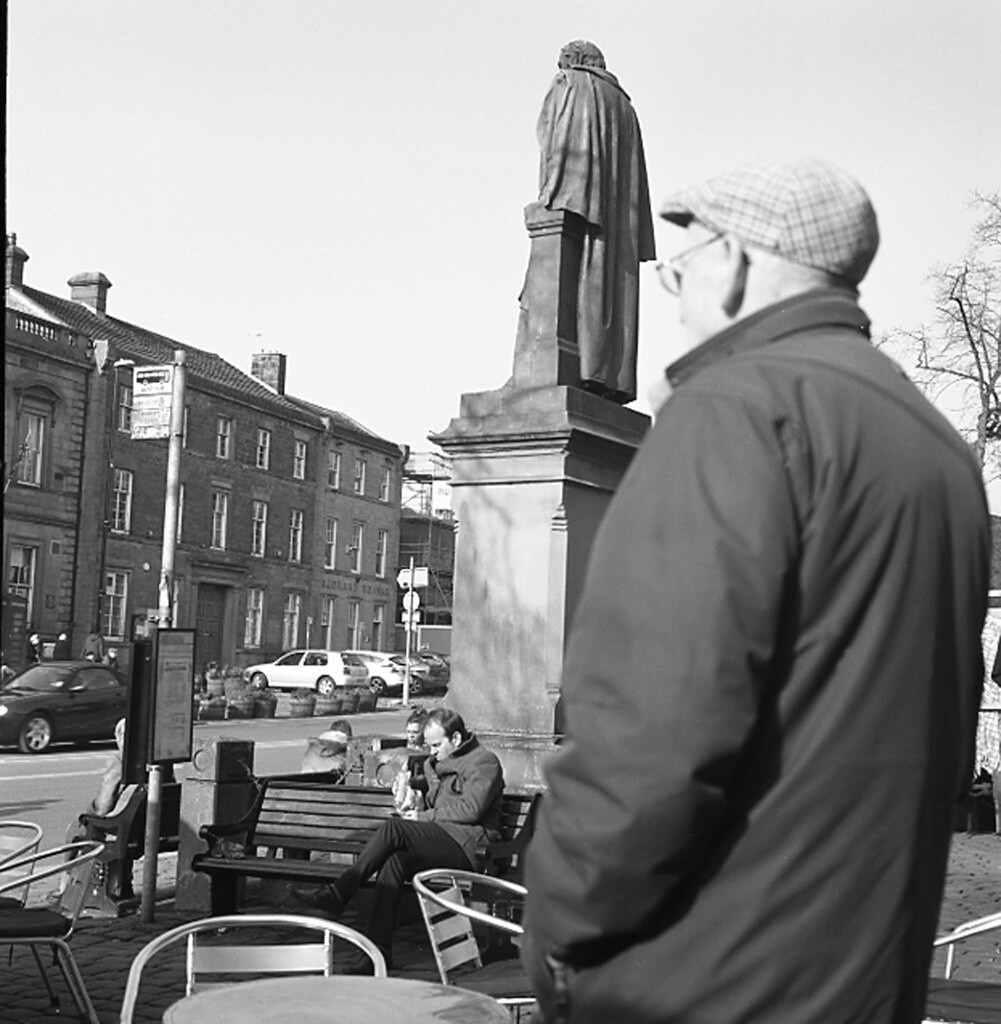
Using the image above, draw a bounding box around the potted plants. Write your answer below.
[192,662,378,721]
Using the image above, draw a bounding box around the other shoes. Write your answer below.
[49,890,63,899]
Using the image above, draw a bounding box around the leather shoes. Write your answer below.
[286,883,346,916]
[342,952,394,975]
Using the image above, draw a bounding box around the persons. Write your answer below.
[285,706,507,974]
[519,154,993,1022]
[497,39,657,407]
[26,625,119,668]
[45,717,175,911]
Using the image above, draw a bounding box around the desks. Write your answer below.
[162,976,513,1024]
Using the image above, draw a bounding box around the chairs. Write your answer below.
[413,868,537,1024]
[0,820,60,1014]
[0,840,104,1024]
[920,912,1001,1024]
[121,914,387,1024]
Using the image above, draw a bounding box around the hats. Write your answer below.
[659,158,879,284]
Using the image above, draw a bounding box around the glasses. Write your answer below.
[655,233,726,296]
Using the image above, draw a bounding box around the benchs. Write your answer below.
[192,780,543,967]
[69,771,346,920]
